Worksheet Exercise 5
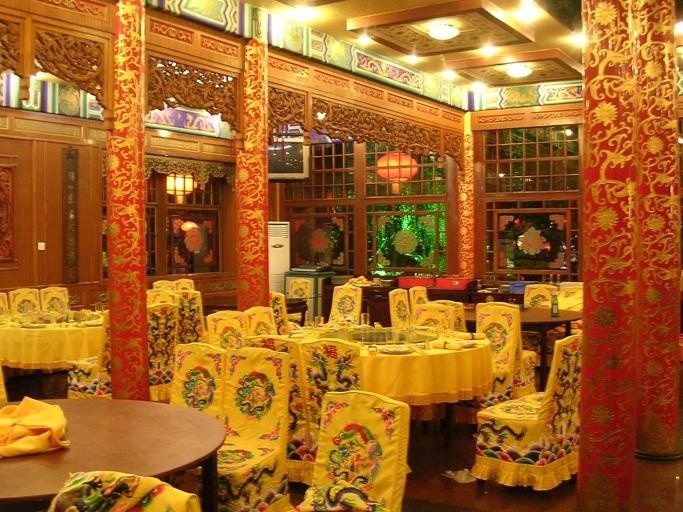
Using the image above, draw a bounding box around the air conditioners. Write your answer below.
[268,220,291,295]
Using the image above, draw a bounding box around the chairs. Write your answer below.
[171,342,224,420]
[205,305,275,347]
[68,310,113,398]
[0,293,8,313]
[148,303,180,401]
[40,286,70,313]
[271,279,583,343]
[290,391,410,510]
[477,328,582,496]
[303,338,365,485]
[8,289,40,313]
[475,301,538,408]
[173,347,290,510]
[148,279,208,345]
[48,470,202,510]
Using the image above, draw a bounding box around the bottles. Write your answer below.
[551,289,560,317]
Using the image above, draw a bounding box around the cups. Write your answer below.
[314,317,324,328]
[360,313,370,327]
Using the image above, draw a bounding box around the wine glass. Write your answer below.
[95,302,110,323]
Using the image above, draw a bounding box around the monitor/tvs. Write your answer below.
[266,138,310,180]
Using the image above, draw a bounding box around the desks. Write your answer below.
[1,396,225,508]
